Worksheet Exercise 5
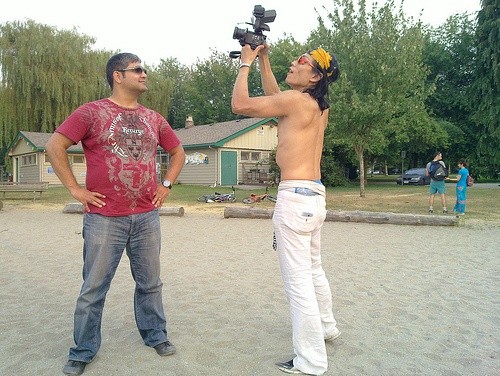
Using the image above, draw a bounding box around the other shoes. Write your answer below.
[324,332,342,343]
[458,213,464,215]
[274,360,302,373]
[442,210,448,214]
[154,341,176,356]
[62,360,86,376]
[429,210,433,214]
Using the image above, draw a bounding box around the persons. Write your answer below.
[445,158,470,215]
[232,47,342,376]
[426,151,448,214]
[45,53,186,376]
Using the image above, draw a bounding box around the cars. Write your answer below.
[397,167,430,186]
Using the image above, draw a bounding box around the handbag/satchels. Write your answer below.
[467,176,474,186]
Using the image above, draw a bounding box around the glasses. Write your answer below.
[298,55,323,78]
[112,65,148,75]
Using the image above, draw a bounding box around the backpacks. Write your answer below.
[429,159,448,181]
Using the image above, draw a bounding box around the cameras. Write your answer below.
[233,4,276,57]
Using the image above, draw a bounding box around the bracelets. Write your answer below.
[239,64,250,68]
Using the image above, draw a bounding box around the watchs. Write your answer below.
[161,178,173,189]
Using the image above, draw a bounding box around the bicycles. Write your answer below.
[197,185,238,203]
[243,187,277,204]
